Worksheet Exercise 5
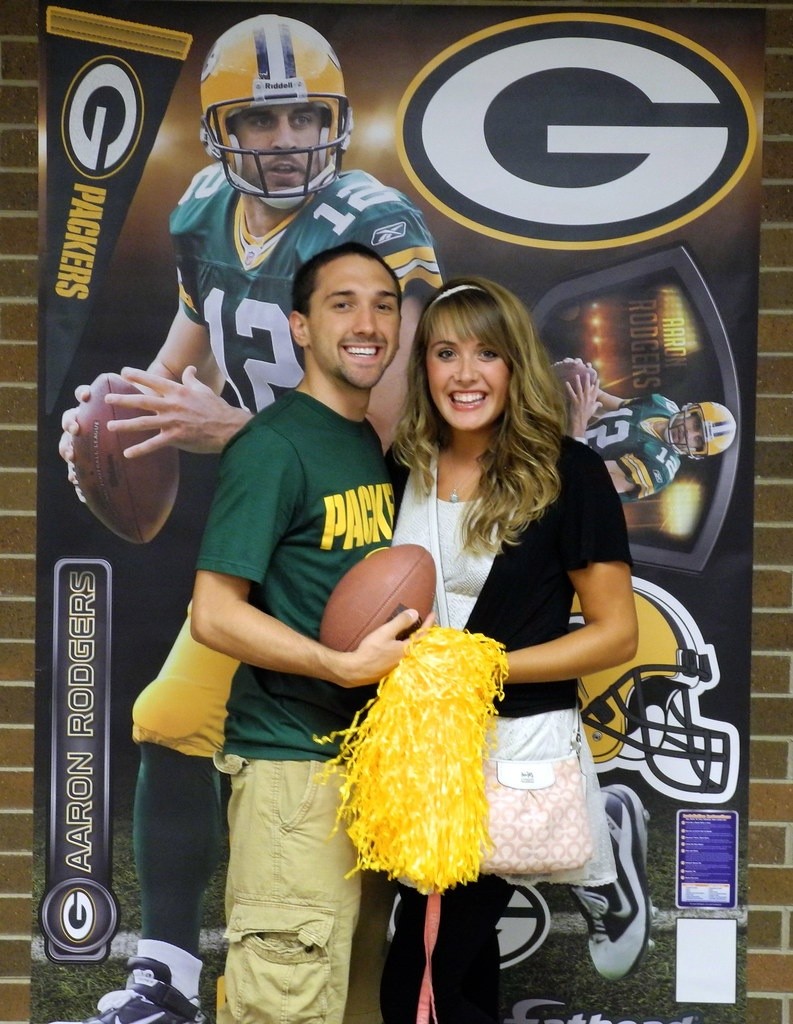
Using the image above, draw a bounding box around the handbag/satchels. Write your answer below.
[478,754,594,874]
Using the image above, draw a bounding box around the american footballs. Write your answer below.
[71,372,180,544]
[319,544,437,652]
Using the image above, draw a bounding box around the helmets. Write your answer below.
[664,402,737,462]
[200,14,352,210]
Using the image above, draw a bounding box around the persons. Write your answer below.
[380,277,639,1024]
[58,13,650,1024]
[553,358,736,503]
[189,241,438,1024]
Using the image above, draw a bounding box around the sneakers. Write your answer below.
[48,956,211,1024]
[567,784,660,983]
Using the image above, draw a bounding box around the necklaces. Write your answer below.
[448,463,474,502]
